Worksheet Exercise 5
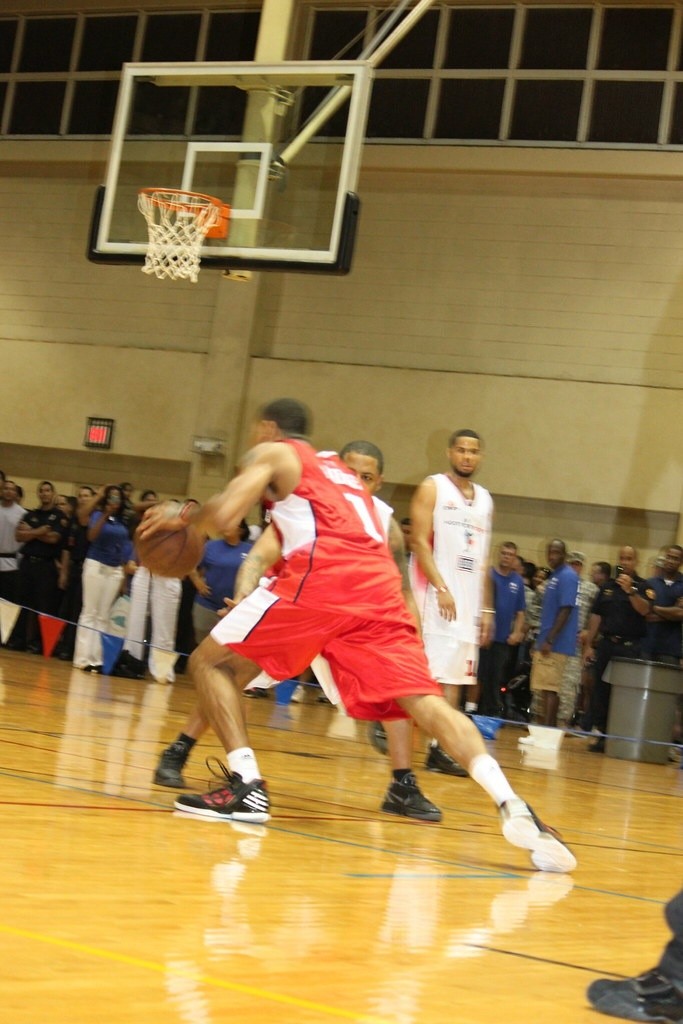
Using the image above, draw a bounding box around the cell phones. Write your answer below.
[615,566,624,578]
[111,496,118,506]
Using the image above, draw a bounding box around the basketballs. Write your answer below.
[137,524,205,577]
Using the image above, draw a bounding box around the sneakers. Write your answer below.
[499,799,577,873]
[383,772,443,820]
[426,744,469,776]
[173,756,271,824]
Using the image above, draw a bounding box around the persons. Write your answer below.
[362,426,497,779]
[134,397,580,878]
[400,517,415,564]
[0,471,332,705]
[587,890,682,1024]
[454,534,683,762]
[149,436,444,820]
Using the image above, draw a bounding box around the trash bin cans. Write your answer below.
[601,656,683,765]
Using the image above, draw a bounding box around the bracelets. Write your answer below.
[434,584,448,594]
[480,608,497,615]
[626,586,637,596]
[175,500,196,527]
[545,636,554,644]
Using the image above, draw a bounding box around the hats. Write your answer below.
[565,550,585,564]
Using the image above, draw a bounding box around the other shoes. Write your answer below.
[587,967,683,1024]
[372,721,388,756]
[153,749,184,788]
